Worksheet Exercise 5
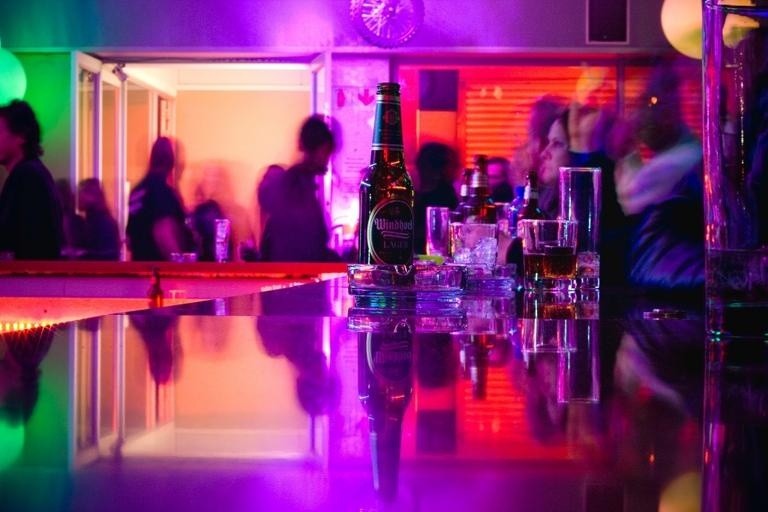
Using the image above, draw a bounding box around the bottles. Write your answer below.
[451,167,471,257]
[464,154,498,268]
[508,187,523,237]
[517,170,547,278]
[359,82,418,294]
[356,331,410,499]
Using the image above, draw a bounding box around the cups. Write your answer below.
[559,165,600,287]
[450,301,599,407]
[450,224,498,273]
[425,207,449,259]
[522,219,575,290]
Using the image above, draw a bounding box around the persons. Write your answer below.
[51,175,90,261]
[417,330,467,390]
[125,135,198,262]
[1,95,72,269]
[407,65,768,317]
[66,178,123,263]
[248,273,347,418]
[610,312,739,440]
[126,306,186,387]
[184,198,236,264]
[250,110,351,261]
[2,325,61,425]
[515,355,589,450]
